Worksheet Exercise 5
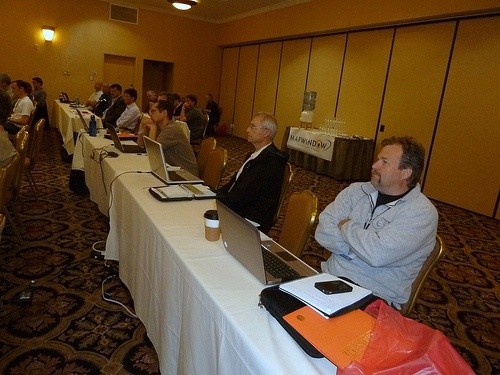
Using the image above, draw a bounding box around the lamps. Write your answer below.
[41,26,55,43]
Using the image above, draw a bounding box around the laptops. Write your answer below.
[76,108,105,132]
[215,199,319,287]
[105,121,147,154]
[143,135,205,186]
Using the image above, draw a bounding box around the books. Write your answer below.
[279,273,372,319]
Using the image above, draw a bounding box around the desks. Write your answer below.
[70,129,476,375]
[138,111,190,144]
[51,99,103,155]
[281,126,373,181]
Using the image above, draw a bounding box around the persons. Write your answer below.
[138,100,198,177]
[215,112,289,235]
[315,135,438,310]
[0,74,222,168]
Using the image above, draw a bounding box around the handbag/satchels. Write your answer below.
[336,300,476,375]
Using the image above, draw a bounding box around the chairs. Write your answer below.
[0,118,46,239]
[278,190,318,258]
[132,109,228,190]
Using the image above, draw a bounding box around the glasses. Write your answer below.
[248,122,261,131]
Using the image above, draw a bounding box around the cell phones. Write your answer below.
[314,280,352,294]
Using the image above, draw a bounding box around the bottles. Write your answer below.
[303,91,317,111]
[89,115,97,137]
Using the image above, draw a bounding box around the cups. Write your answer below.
[203,210,221,241]
